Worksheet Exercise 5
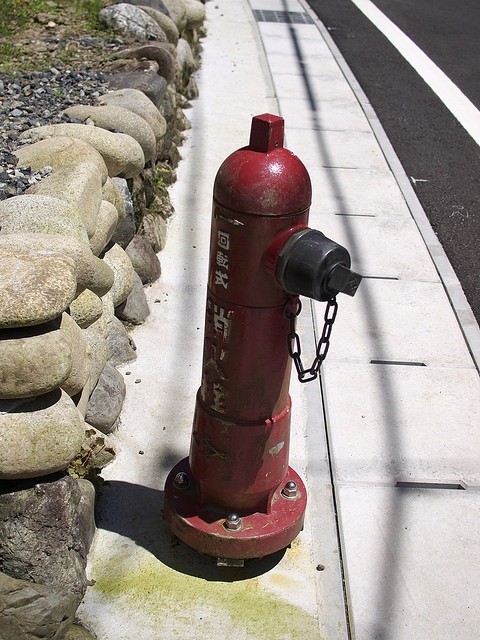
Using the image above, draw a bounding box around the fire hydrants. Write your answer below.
[160,110,363,561]
